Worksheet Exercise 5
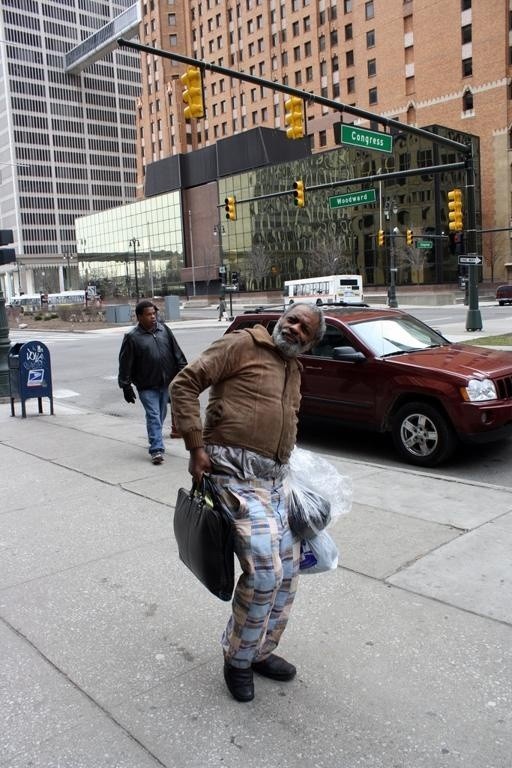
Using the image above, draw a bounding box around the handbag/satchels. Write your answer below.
[173,478,235,601]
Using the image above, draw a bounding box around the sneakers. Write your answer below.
[151,450,163,463]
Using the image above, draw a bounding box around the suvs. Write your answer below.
[220,307,512,468]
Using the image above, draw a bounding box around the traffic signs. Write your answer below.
[338,123,393,154]
[328,190,378,210]
[417,240,434,251]
[458,255,482,266]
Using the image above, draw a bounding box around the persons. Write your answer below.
[222,297,227,307]
[117,301,189,463]
[167,301,327,702]
[216,297,225,321]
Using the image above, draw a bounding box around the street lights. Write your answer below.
[213,221,225,266]
[129,236,140,304]
[14,260,26,291]
[384,200,397,307]
[63,251,75,290]
[41,271,46,294]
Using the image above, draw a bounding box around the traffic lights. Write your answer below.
[377,229,386,248]
[225,195,238,222]
[283,86,309,140]
[180,65,207,126]
[292,180,306,209]
[446,190,464,233]
[406,229,415,246]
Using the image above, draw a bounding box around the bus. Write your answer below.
[8,296,42,315]
[47,292,87,313]
[283,275,364,312]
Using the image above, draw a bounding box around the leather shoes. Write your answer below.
[224,658,254,701]
[253,654,295,681]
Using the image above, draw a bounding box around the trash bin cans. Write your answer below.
[7,341,53,419]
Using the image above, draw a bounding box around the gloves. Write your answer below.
[123,385,136,403]
